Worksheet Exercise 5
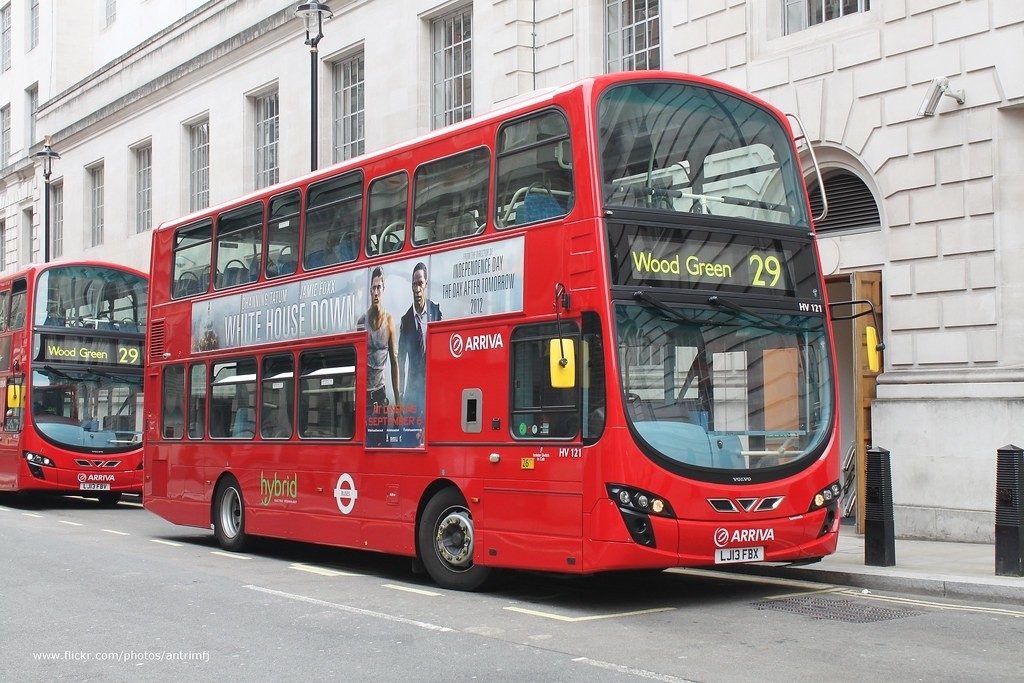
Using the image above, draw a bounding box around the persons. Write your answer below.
[397,262,442,448]
[356,266,401,448]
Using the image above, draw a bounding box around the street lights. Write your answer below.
[32,134,63,262]
[294,0,336,174]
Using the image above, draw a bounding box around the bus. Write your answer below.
[142,70,886,592]
[0,257,150,507]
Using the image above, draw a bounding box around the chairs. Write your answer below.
[171,227,402,300]
[1,310,137,331]
